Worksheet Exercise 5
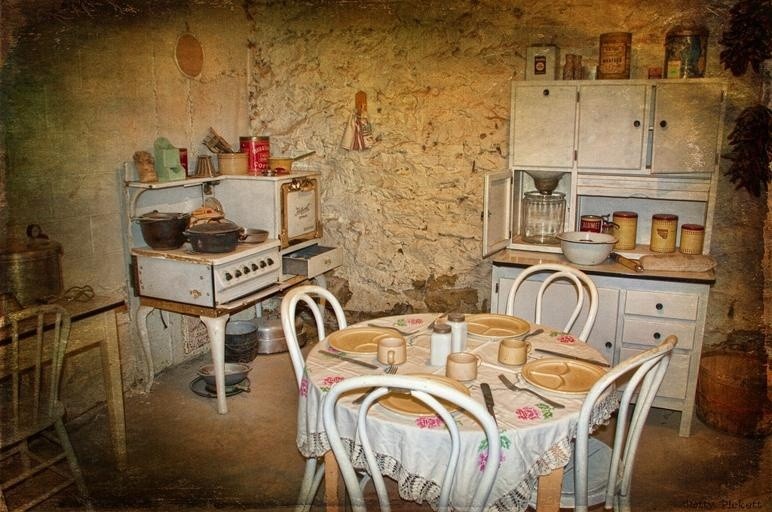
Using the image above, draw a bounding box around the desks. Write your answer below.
[1,286,135,481]
[134,273,331,416]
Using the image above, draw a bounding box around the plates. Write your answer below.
[374,373,472,416]
[522,358,609,395]
[464,313,531,339]
[326,326,402,354]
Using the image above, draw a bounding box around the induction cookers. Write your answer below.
[129,241,281,292]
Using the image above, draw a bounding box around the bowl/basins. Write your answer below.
[238,227,269,243]
[555,231,618,266]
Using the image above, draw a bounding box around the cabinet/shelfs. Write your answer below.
[575,78,727,174]
[213,170,343,280]
[481,78,578,262]
[489,248,719,439]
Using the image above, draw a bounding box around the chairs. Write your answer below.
[504,261,598,342]
[276,282,353,512]
[1,301,96,512]
[321,374,503,512]
[524,331,678,512]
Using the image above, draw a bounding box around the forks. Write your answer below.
[367,323,419,336]
[352,364,399,404]
[498,375,565,409]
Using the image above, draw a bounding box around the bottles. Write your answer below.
[195,155,215,178]
[429,312,468,368]
[664,19,710,78]
[521,191,567,244]
[601,222,615,259]
[562,53,583,81]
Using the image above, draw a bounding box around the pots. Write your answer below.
[268,149,317,172]
[0,236,64,309]
[198,316,308,393]
[129,208,190,251]
[184,215,248,255]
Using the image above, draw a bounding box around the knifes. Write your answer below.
[480,382,498,426]
[428,309,458,329]
[318,350,378,369]
[534,348,611,368]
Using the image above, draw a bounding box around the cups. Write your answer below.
[376,337,408,366]
[497,338,532,366]
[445,352,483,381]
